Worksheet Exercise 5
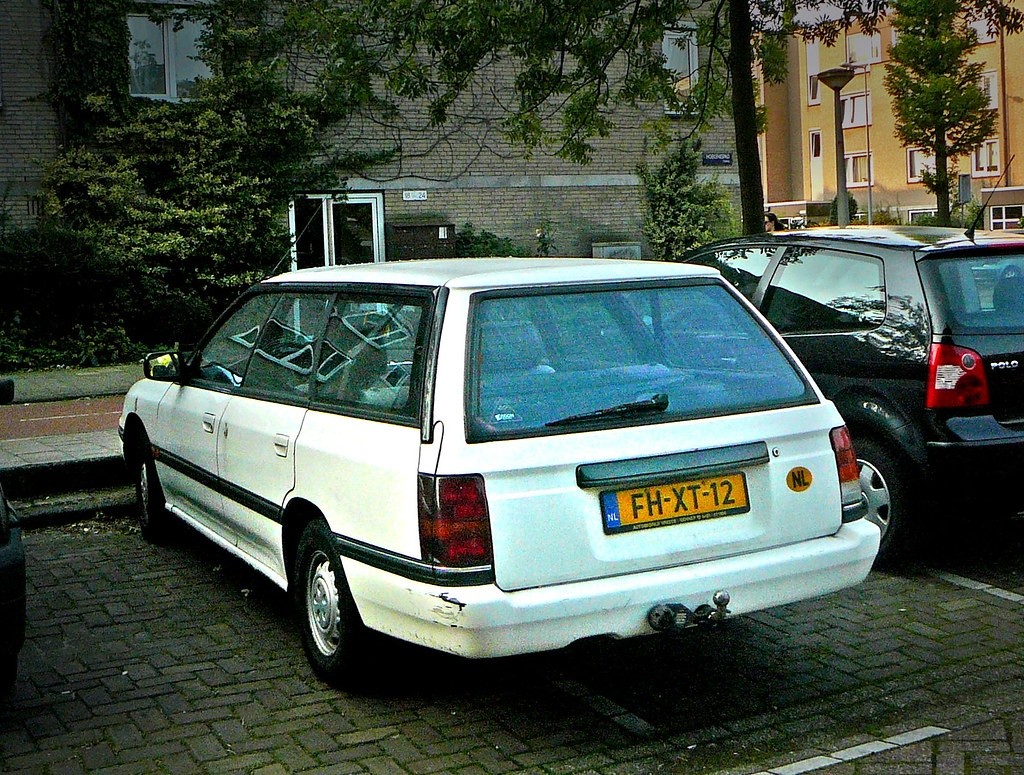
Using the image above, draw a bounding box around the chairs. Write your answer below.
[480,318,552,378]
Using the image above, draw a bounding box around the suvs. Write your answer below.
[629,224,1024,573]
[116,258,882,679]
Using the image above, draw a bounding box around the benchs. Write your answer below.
[477,363,687,429]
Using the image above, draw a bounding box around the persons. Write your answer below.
[764,213,785,232]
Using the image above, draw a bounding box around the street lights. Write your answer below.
[817,67,855,226]
[841,62,873,225]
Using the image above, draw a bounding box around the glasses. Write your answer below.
[764,221,770,224]
